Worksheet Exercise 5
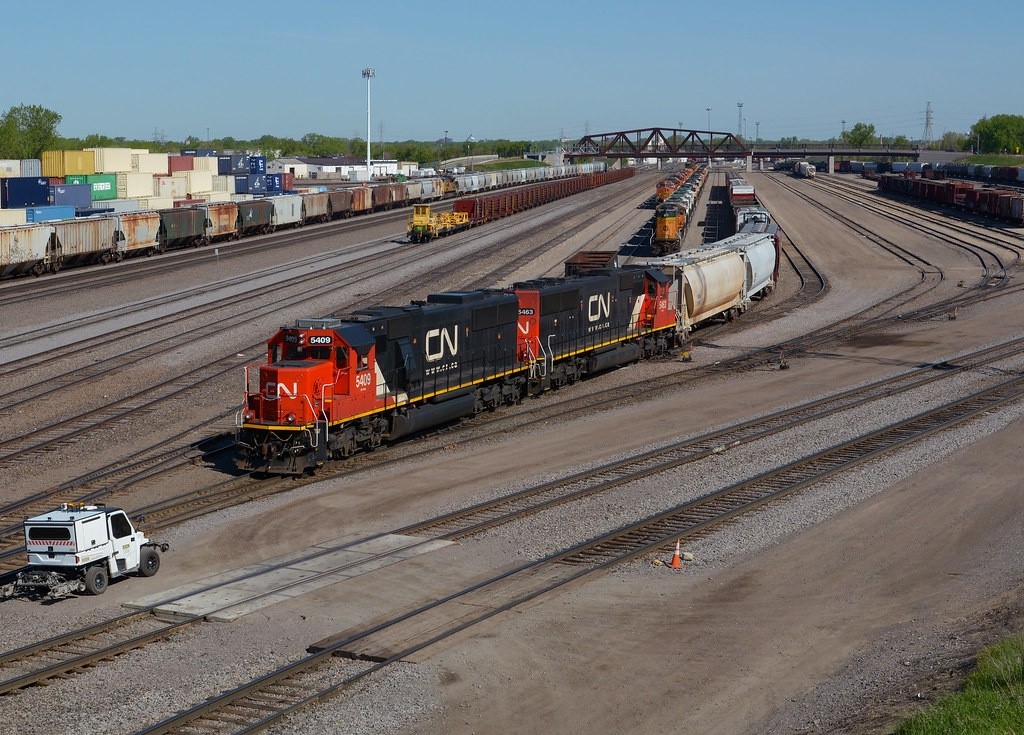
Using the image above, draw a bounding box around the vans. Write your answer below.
[14,499,161,602]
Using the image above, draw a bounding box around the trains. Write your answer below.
[787,158,816,179]
[229,166,781,496]
